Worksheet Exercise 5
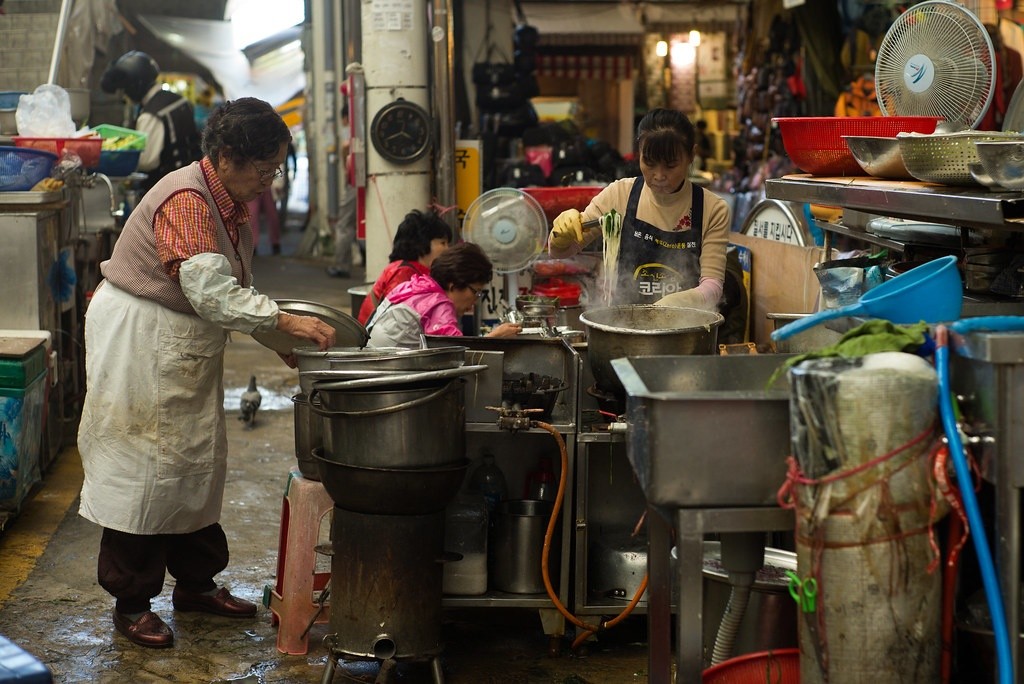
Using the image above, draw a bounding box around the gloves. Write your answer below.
[653,287,705,312]
[549,207,590,248]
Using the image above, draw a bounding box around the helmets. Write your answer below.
[115,50,160,102]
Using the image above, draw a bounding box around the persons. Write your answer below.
[77,97,337,648]
[248,137,297,257]
[547,108,730,313]
[357,207,452,335]
[364,242,523,355]
[106,49,203,195]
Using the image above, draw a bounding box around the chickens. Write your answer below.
[236,376,263,425]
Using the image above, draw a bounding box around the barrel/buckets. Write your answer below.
[492,498,559,595]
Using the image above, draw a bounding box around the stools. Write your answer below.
[262,465,334,656]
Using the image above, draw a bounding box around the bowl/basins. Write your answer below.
[974,140,1024,193]
[840,134,917,180]
[515,380,570,420]
[966,162,1015,194]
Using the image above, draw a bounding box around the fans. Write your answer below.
[462,188,549,311]
[875,1,997,130]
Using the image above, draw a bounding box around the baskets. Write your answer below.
[520,187,606,214]
[771,112,949,176]
[0,121,148,192]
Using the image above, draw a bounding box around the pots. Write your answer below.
[701,560,804,665]
[291,344,468,516]
[580,300,725,405]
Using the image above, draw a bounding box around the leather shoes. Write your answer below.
[112,603,174,647]
[172,583,258,616]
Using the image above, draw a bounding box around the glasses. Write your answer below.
[247,157,287,184]
[462,281,483,302]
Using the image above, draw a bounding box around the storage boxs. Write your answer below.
[518,186,606,223]
[10,137,106,168]
[0,345,49,513]
[91,124,149,151]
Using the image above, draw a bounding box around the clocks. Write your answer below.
[371,99,434,164]
